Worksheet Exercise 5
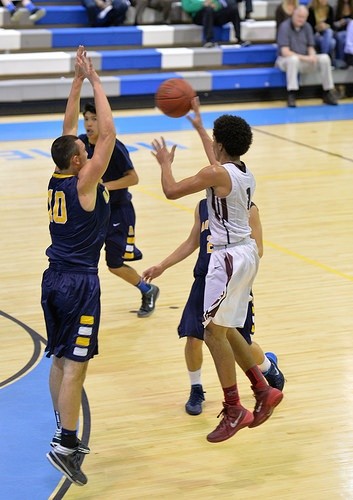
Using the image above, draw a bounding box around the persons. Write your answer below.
[40,46,117,487]
[151,96,284,443]
[182,0,250,47]
[76,104,160,318]
[83,0,256,26]
[141,198,284,415]
[1,0,46,22]
[277,6,343,108]
[274,0,353,70]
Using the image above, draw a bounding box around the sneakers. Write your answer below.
[137,284,159,318]
[50,428,90,454]
[185,384,206,415]
[207,402,254,443]
[46,445,88,486]
[262,352,287,391]
[248,385,284,428]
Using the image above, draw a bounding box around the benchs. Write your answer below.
[0,0,353,103]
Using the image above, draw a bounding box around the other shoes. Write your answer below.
[288,94,295,106]
[323,91,337,105]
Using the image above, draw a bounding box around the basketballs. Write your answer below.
[156,78,195,118]
